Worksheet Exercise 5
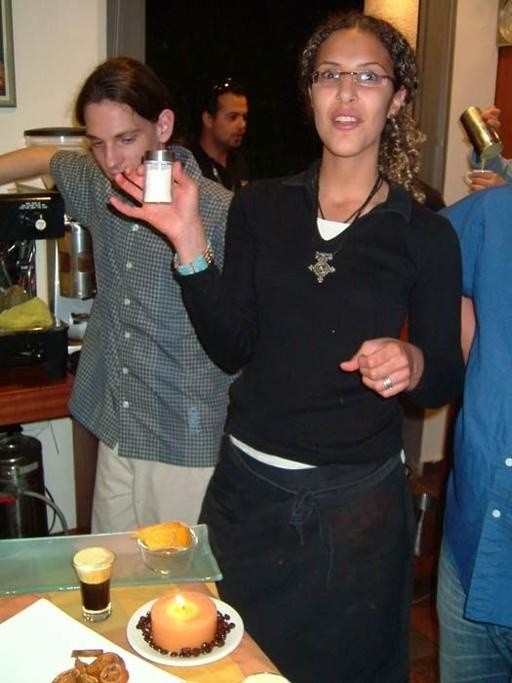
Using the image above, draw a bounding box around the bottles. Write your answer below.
[142,149,176,203]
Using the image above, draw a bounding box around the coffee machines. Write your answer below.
[0,182,72,391]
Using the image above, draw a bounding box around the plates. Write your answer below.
[0,523,226,598]
[0,598,191,681]
[126,587,245,667]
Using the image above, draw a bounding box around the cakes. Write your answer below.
[132,522,193,554]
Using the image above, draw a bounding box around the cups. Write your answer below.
[458,104,506,162]
[74,548,115,623]
[461,168,496,191]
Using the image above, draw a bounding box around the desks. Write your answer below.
[0,527,290,683]
[0,370,98,533]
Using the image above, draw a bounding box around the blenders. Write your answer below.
[21,126,103,342]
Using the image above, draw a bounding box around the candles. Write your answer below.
[152,588,217,654]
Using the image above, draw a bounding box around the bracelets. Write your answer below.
[172,240,214,275]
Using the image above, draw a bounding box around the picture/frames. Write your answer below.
[0,0,17,107]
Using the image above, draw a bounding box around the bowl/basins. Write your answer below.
[134,520,200,577]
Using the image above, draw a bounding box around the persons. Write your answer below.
[423,183,512,682]
[109,14,466,682]
[180,78,249,191]
[0,54,235,535]
[461,103,512,194]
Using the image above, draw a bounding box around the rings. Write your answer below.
[383,376,392,389]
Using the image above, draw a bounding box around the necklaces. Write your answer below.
[308,166,385,284]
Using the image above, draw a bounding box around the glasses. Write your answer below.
[309,67,397,88]
